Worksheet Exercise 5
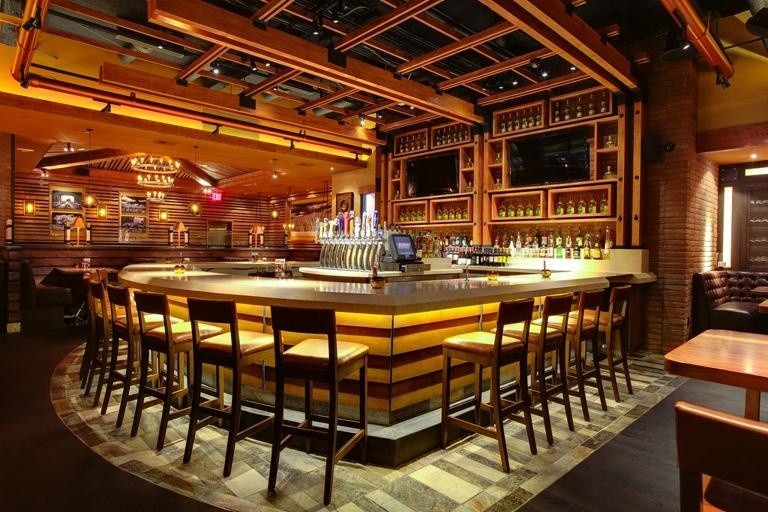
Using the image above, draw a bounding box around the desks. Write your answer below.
[39,266,119,325]
[664,327,767,423]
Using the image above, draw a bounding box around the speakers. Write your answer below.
[664,17,696,62]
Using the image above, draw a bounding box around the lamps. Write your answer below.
[130,154,182,204]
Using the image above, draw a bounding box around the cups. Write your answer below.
[251,253,258,262]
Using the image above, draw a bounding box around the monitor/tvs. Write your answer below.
[389,234,417,261]
[406,152,459,197]
[508,131,590,186]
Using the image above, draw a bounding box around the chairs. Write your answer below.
[530,288,609,422]
[438,294,540,474]
[485,293,578,445]
[180,295,286,480]
[263,302,370,507]
[673,400,767,512]
[129,289,225,451]
[565,283,633,406]
[80,266,186,430]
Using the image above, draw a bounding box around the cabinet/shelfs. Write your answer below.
[386,120,480,246]
[481,84,626,247]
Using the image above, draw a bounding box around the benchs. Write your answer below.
[20,259,71,332]
[691,269,767,337]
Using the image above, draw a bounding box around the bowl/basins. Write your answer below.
[174,265,186,274]
[488,271,501,280]
[541,269,551,278]
[370,276,385,289]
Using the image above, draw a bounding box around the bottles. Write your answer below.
[510,224,612,259]
[398,207,467,221]
[465,153,502,189]
[606,135,614,147]
[501,90,606,132]
[398,122,469,153]
[497,192,607,216]
[411,229,510,267]
[604,164,613,179]
